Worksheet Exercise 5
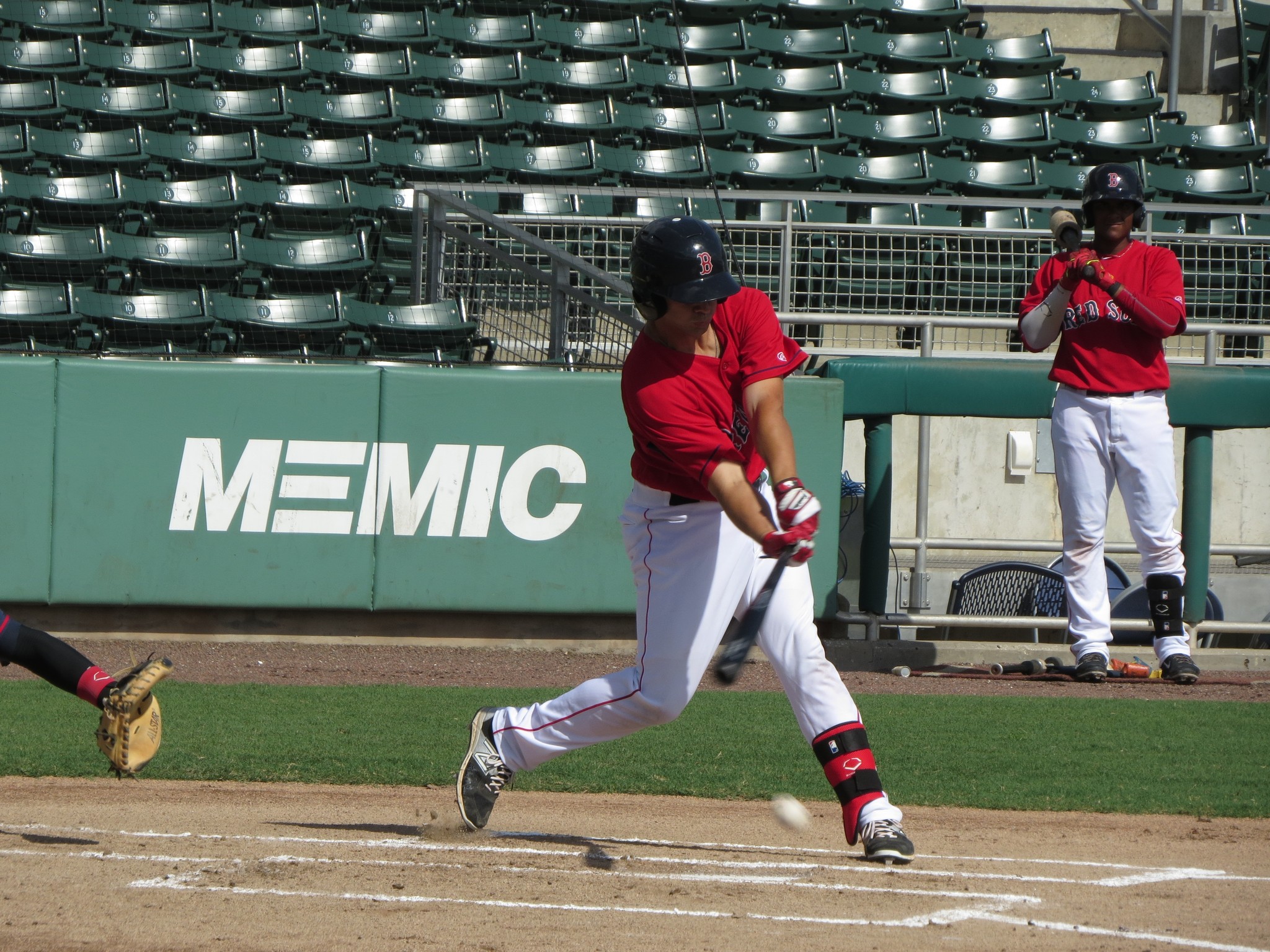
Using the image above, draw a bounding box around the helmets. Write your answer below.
[630,215,741,320]
[1081,162,1146,228]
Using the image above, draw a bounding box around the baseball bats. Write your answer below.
[1049,205,1095,279]
[713,548,793,687]
[988,656,1065,675]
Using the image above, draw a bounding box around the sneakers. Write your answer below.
[1160,652,1199,682]
[1075,653,1108,680]
[456,706,516,831]
[860,819,914,860]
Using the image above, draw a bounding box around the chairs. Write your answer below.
[0,0,1270,370]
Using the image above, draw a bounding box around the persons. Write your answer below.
[453,216,919,862]
[1020,162,1202,684]
[0,611,176,781]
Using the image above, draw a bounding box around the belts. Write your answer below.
[1086,389,1154,397]
[669,494,699,506]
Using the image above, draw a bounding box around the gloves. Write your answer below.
[774,477,822,538]
[1058,247,1115,291]
[763,531,814,567]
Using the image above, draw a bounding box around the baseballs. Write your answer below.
[773,792,809,834]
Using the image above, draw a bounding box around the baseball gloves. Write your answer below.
[94,655,174,775]
